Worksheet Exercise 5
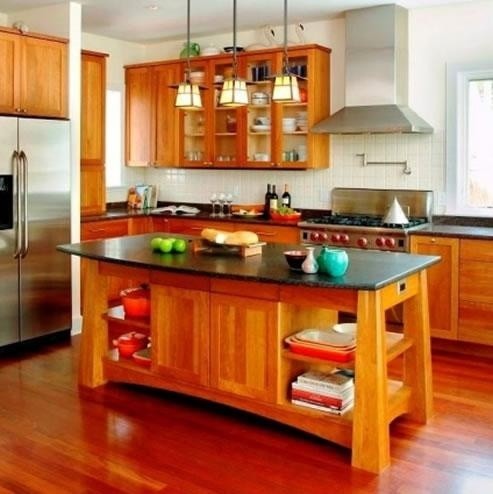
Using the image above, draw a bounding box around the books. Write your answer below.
[151,204,201,214]
[289,365,355,416]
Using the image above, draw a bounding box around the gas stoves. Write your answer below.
[298,211,428,233]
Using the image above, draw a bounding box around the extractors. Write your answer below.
[306,0,436,135]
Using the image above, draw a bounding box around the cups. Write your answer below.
[282,150,299,161]
[186,150,204,161]
[216,151,236,161]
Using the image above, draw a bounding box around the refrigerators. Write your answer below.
[0,114,72,360]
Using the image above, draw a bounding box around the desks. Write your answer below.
[56,232,443,472]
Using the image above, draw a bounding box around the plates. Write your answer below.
[189,71,205,81]
[231,211,263,218]
[223,46,243,52]
[331,321,357,335]
[282,327,357,362]
[249,124,270,131]
[281,110,307,131]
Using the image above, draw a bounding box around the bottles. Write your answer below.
[281,182,291,208]
[300,243,347,276]
[264,183,271,214]
[272,184,277,210]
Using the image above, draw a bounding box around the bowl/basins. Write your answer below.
[251,90,269,104]
[255,153,267,161]
[283,249,309,269]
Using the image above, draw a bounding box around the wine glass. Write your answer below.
[225,191,234,216]
[217,191,225,216]
[208,191,218,216]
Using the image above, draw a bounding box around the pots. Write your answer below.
[119,287,150,317]
[111,330,147,358]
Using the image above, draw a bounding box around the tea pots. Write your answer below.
[382,195,411,224]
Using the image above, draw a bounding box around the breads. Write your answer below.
[201,229,258,245]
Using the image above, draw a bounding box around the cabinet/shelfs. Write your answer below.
[242,47,330,169]
[156,217,233,238]
[233,221,300,245]
[123,62,180,168]
[0,28,63,118]
[128,216,154,235]
[457,238,491,346]
[180,56,242,169]
[409,234,458,342]
[80,219,128,242]
[81,51,104,165]
[81,165,106,217]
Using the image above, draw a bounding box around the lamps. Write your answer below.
[173,0,204,110]
[217,1,249,108]
[271,0,301,104]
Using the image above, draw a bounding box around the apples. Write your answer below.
[151,238,186,253]
